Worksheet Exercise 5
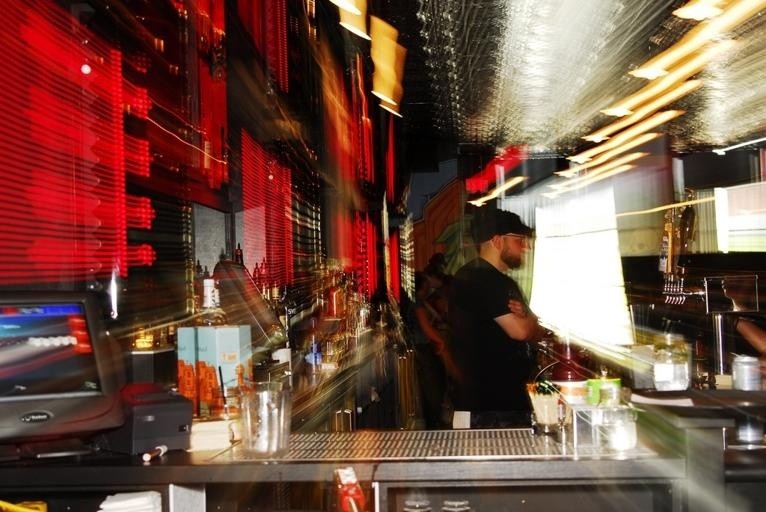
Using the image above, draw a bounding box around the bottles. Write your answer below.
[655,335,689,389]
[195,241,232,278]
[312,258,370,356]
[234,243,243,266]
[252,257,286,328]
[731,357,762,444]
[132,318,175,348]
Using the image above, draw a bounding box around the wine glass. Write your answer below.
[377,302,386,328]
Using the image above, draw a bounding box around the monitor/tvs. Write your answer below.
[0,290,126,440]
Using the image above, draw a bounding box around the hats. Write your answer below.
[472,208,536,244]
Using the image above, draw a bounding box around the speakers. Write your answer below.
[682,151,761,191]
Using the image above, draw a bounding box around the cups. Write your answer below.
[224,385,293,457]
[596,405,638,460]
[585,377,622,410]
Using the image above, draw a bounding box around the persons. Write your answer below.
[388,207,540,428]
[720,276,765,360]
